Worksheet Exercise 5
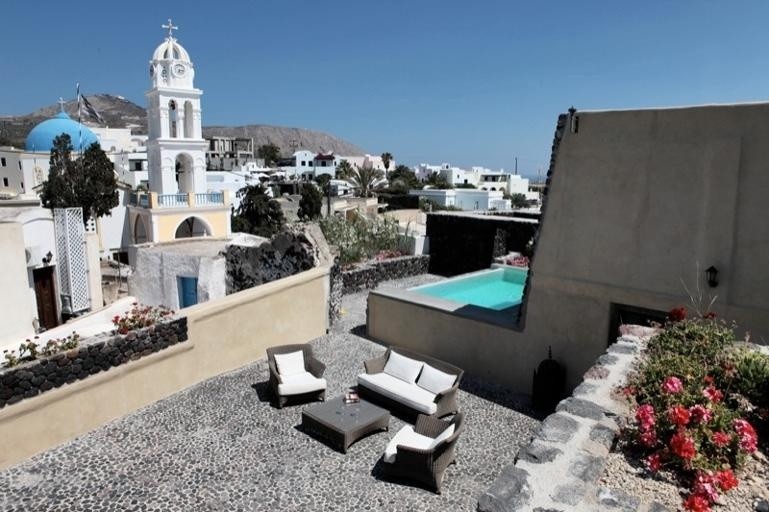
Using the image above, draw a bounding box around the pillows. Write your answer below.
[273,349,305,378]
[429,422,455,449]
[384,350,457,394]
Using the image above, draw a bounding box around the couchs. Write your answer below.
[358,345,464,419]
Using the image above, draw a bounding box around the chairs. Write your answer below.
[382,412,464,494]
[266,343,327,409]
